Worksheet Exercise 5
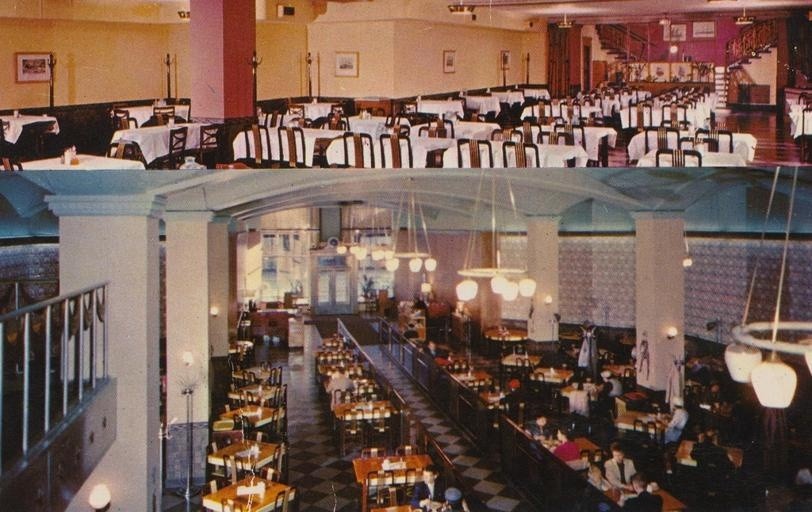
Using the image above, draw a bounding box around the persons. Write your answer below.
[404,323,419,339]
[325,367,355,412]
[702,382,723,405]
[730,394,756,452]
[635,340,650,386]
[411,296,428,313]
[441,487,465,512]
[587,464,612,492]
[410,464,446,508]
[321,351,336,365]
[591,370,624,417]
[608,332,629,365]
[524,413,553,441]
[423,340,441,359]
[663,396,689,445]
[604,442,636,491]
[623,474,663,512]
[553,425,580,462]
[499,379,527,419]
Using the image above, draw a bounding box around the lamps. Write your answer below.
[455,169,536,301]
[659,14,671,25]
[337,177,437,273]
[682,222,693,267]
[448,0,475,13]
[558,14,573,29]
[722,165,811,410]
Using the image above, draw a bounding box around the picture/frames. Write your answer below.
[663,24,687,42]
[443,48,456,74]
[692,20,715,39]
[499,49,512,69]
[14,51,52,84]
[332,51,359,79]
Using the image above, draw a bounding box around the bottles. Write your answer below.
[64,146,76,165]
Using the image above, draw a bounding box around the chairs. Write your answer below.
[201,331,300,512]
[0,158,22,170]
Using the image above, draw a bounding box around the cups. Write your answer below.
[13,110,18,117]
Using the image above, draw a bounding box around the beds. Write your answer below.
[0,153,143,170]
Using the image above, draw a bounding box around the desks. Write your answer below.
[785,91,812,163]
